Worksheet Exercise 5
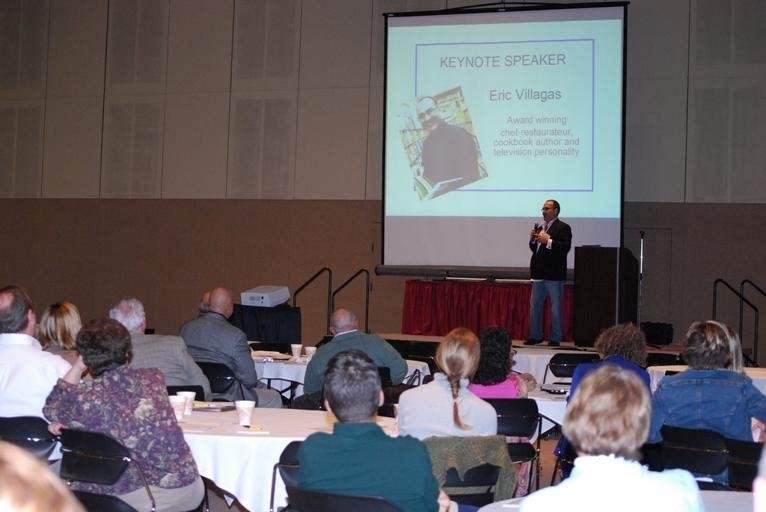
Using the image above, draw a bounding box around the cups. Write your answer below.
[304,347,317,361]
[168,395,187,422]
[235,401,256,427]
[176,391,196,416]
[291,344,303,359]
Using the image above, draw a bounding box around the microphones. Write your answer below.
[530,222,540,242]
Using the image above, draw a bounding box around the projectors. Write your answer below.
[241,285,291,307]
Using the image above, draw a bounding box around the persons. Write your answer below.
[395,328,498,439]
[553,323,652,480]
[475,364,707,512]
[645,319,766,490]
[302,309,407,412]
[466,326,529,444]
[523,200,572,345]
[751,444,766,512]
[279,350,465,511]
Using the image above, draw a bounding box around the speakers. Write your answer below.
[574,246,638,347]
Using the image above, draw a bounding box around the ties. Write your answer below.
[544,225,548,232]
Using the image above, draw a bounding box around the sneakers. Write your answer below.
[523,338,543,345]
[547,340,560,346]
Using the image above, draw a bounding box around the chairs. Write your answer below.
[166,339,766,511]
[0,415,208,512]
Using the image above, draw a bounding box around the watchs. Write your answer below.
[414,95,482,192]
